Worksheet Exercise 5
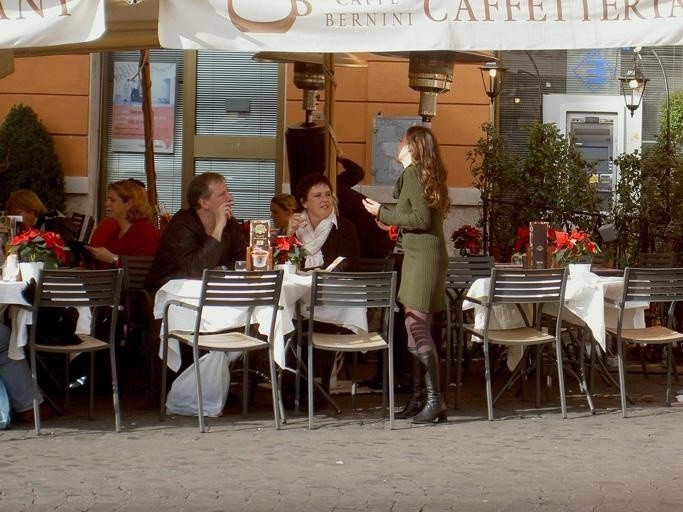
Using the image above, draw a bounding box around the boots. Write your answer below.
[391,344,449,423]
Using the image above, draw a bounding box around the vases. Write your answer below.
[21,262,44,283]
[569,264,592,275]
[274,258,298,275]
[512,252,526,265]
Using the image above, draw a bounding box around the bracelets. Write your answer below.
[220,265,228,276]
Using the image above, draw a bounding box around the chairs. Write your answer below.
[585,266,683,419]
[71,214,84,242]
[158,267,285,434]
[445,256,496,367]
[451,267,571,422]
[115,255,160,395]
[31,261,127,433]
[291,268,398,432]
[84,215,95,244]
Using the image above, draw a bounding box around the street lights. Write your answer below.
[618,47,670,211]
[477,50,543,123]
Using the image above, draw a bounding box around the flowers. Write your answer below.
[11,231,66,261]
[275,234,304,264]
[516,227,557,257]
[557,229,601,263]
[451,225,490,253]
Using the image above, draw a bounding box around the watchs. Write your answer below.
[111,253,119,265]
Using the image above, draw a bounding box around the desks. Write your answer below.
[153,277,374,374]
[461,278,653,372]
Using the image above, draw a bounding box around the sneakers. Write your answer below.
[17,405,54,421]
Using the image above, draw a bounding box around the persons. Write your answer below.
[270,194,296,236]
[144,172,250,407]
[362,126,450,423]
[5,189,57,232]
[0,240,53,423]
[69,178,161,392]
[278,173,361,410]
[337,148,414,394]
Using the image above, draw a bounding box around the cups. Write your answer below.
[251,249,269,267]
[298,214,312,234]
[235,261,247,271]
[2,265,12,282]
